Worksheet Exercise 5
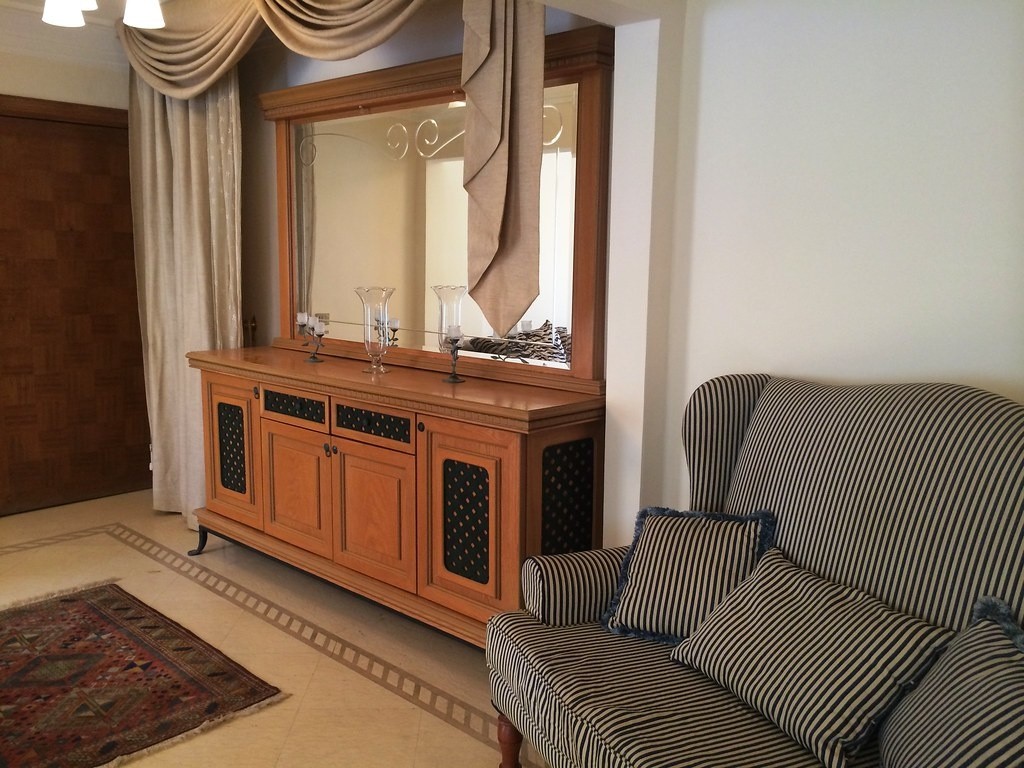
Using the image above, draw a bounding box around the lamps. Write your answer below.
[41,0,166,30]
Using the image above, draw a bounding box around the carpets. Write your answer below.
[0,578,295,768]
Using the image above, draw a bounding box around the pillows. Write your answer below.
[876,595,1024,768]
[600,505,778,646]
[667,543,958,768]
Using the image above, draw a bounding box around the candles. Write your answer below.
[297,311,308,323]
[493,329,500,339]
[309,316,318,327]
[448,325,462,338]
[314,322,325,332]
[390,318,400,328]
[507,324,517,335]
[520,320,533,332]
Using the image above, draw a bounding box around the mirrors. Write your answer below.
[253,23,614,399]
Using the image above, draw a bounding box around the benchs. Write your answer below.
[486,374,1024,768]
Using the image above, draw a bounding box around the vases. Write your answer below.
[354,286,396,374]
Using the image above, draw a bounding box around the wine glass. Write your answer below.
[354,288,395,373]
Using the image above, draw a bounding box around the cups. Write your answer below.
[431,284,468,354]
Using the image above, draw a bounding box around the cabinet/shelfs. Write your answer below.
[183,347,606,653]
